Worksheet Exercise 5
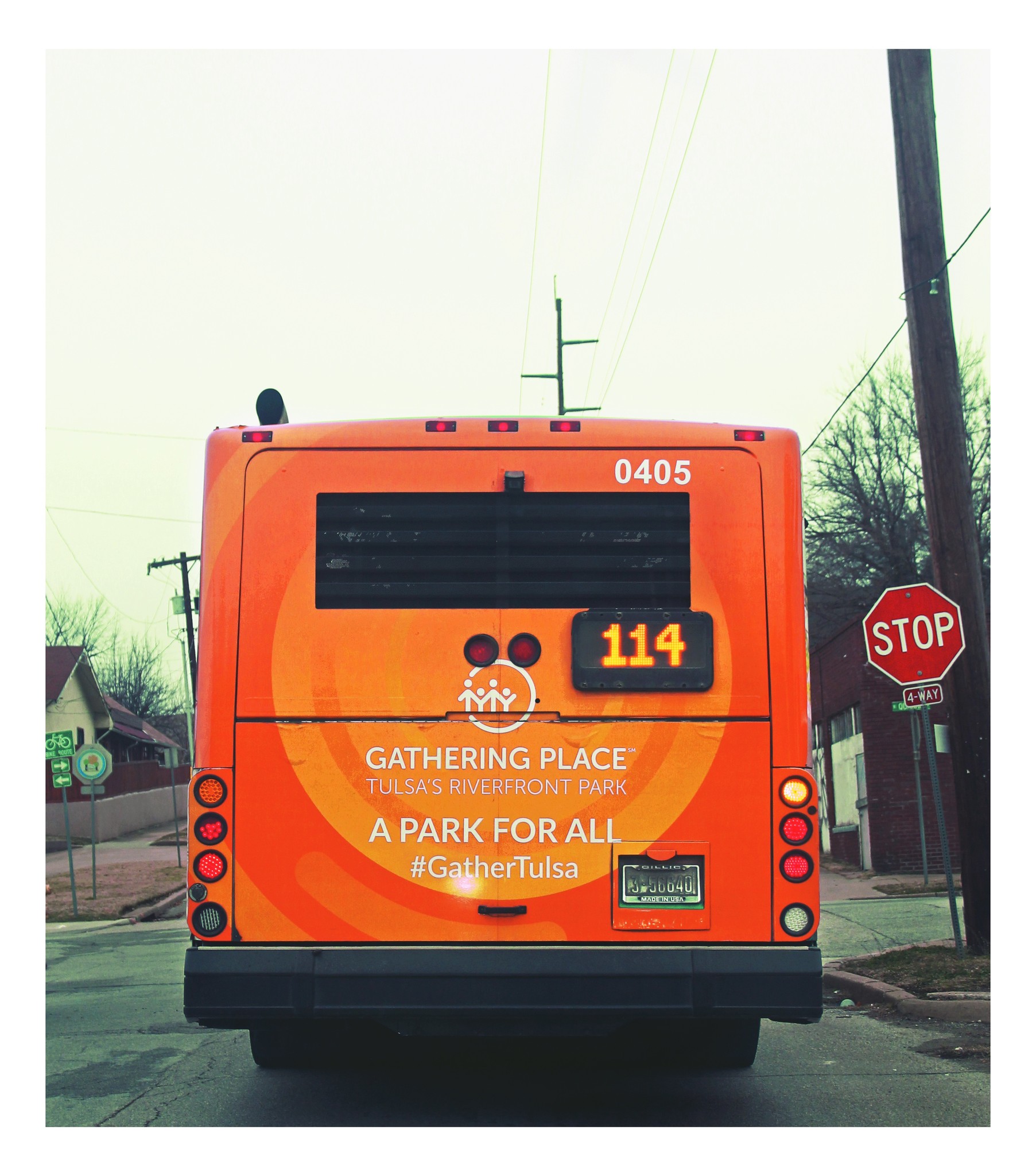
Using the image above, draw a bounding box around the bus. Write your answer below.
[182,388,825,1077]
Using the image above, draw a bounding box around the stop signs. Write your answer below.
[863,582,966,685]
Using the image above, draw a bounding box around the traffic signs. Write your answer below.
[903,684,943,707]
[892,701,931,712]
[51,758,72,788]
[45,729,75,760]
[76,749,106,779]
